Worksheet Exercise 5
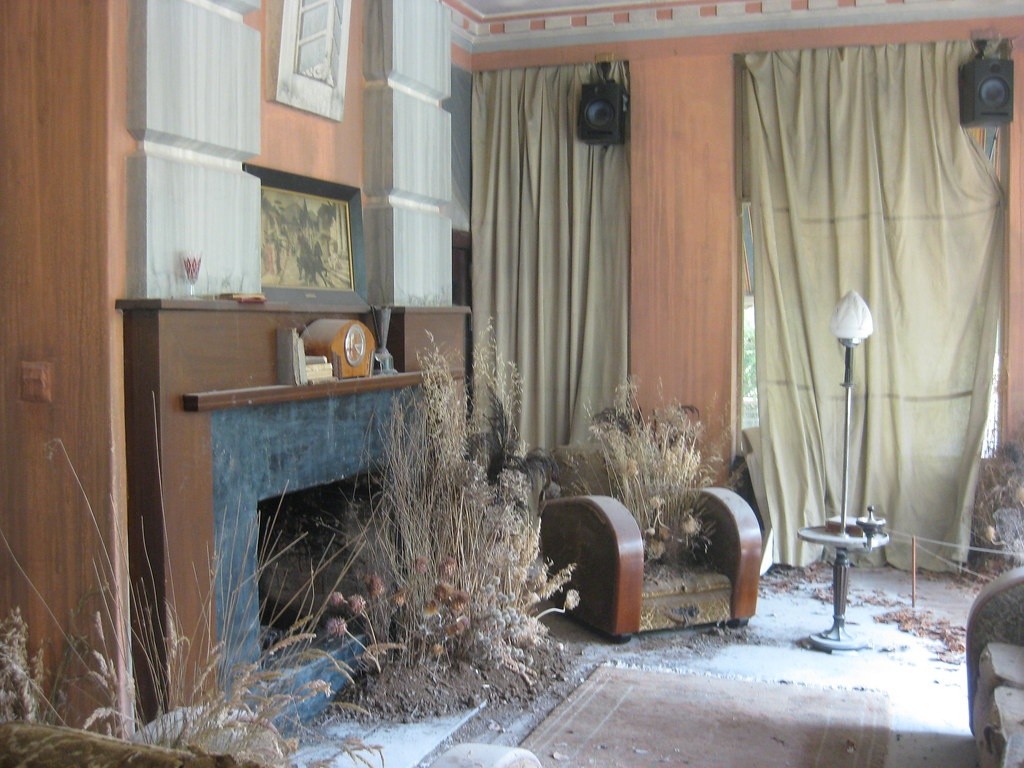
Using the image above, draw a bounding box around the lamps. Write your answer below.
[827,290,874,537]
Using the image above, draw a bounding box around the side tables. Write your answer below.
[796,525,890,651]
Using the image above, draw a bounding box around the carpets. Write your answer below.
[520,661,890,768]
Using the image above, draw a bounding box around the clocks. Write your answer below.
[301,317,374,377]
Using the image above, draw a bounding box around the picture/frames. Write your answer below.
[243,163,368,305]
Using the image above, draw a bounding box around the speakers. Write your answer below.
[578,83,627,145]
[958,56,1014,128]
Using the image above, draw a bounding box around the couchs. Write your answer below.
[539,447,762,643]
[965,566,1024,768]
[0,706,543,768]
